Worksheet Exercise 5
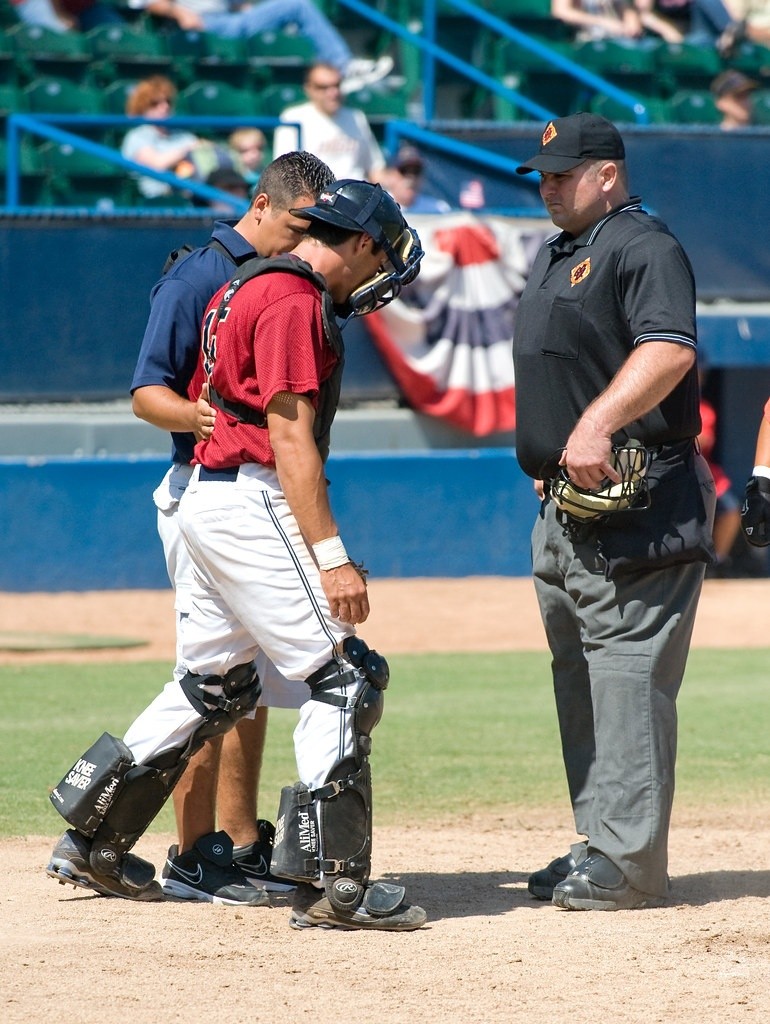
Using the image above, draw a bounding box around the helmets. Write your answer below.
[289,179,405,245]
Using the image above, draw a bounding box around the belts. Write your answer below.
[199,464,240,482]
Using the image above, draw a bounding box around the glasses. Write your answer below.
[394,164,423,174]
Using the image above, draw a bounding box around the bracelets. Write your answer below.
[313,535,349,569]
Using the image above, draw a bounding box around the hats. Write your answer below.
[516,112,626,175]
[386,145,421,168]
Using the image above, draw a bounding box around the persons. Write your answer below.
[513,111,717,907]
[122,78,265,210]
[130,151,336,905]
[15,0,394,94]
[273,65,384,184]
[712,70,758,129]
[48,180,427,930]
[696,352,742,574]
[379,146,453,214]
[550,0,770,55]
[741,397,770,547]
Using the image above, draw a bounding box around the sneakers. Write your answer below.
[45,828,163,902]
[161,830,270,905]
[235,820,298,892]
[552,855,673,910]
[527,847,596,900]
[290,883,427,930]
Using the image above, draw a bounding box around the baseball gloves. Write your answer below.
[347,554,371,588]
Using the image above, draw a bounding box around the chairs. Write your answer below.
[0,0,770,211]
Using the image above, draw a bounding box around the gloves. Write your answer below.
[738,475,770,546]
